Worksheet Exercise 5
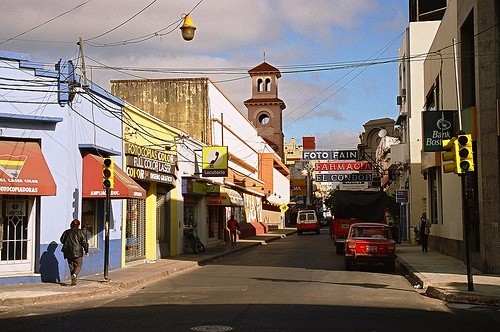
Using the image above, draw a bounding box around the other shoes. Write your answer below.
[71,275,77,285]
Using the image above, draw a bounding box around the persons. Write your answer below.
[228,215,240,245]
[418,212,431,252]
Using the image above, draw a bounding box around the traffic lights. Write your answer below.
[458,133,475,172]
[441,137,461,174]
[101,157,114,189]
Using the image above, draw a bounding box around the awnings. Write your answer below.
[0,140,57,197]
[82,153,146,199]
[207,188,246,207]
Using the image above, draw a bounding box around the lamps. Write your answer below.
[180,15,196,40]
[234,180,281,198]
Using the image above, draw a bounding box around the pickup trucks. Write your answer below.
[345,221,398,271]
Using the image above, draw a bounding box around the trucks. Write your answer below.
[333,190,387,254]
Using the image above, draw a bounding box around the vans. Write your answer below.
[297,210,320,234]
